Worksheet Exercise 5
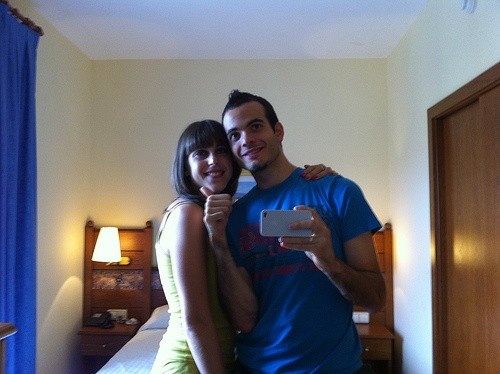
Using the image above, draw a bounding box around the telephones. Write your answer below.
[83,311,111,328]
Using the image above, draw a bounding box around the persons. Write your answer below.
[200,88,387,374]
[149,120,337,374]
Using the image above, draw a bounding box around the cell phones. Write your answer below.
[259,210,313,237]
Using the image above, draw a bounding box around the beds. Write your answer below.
[84,220,392,374]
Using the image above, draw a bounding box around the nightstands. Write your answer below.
[78,319,144,358]
[354,323,394,374]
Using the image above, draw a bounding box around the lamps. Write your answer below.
[91,227,131,266]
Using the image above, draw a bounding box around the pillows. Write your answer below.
[140,304,170,330]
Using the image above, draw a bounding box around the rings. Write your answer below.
[319,164,325,170]
[309,237,313,243]
